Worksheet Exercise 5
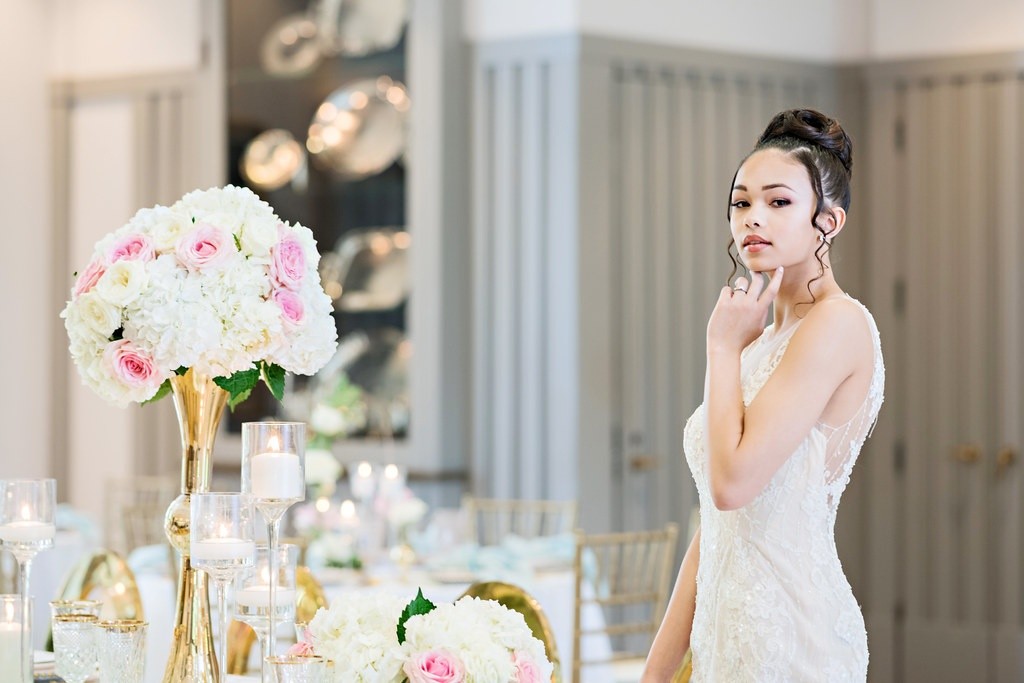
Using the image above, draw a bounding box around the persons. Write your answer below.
[637,109,886,683]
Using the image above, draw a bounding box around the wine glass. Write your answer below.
[234,543,298,683]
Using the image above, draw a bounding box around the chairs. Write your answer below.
[575,524,679,683]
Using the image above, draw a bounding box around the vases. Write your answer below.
[166,365,230,683]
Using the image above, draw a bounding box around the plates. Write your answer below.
[33,648,58,681]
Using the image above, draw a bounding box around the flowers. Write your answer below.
[61,184,339,409]
[289,584,555,683]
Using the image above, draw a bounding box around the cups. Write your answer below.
[49,600,100,682]
[263,653,334,683]
[98,620,148,683]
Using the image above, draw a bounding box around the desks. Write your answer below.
[0,495,98,657]
[126,538,611,683]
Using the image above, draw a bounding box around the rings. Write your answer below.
[734,287,747,293]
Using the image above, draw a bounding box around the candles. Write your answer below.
[0,506,54,541]
[193,523,251,560]
[236,572,287,605]
[251,434,300,499]
[0,602,22,683]
